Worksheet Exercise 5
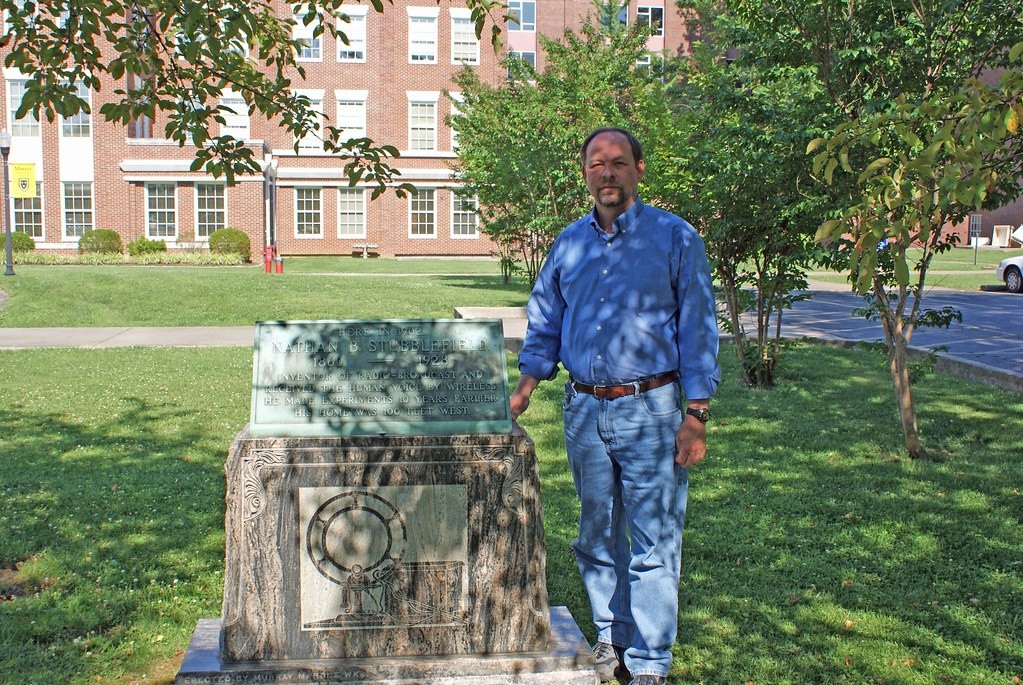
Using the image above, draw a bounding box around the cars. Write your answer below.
[996,255,1023,294]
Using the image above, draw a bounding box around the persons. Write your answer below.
[509,129,720,685]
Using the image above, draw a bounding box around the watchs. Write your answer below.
[686,406,711,423]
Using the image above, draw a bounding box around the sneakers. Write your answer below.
[593,640,623,682]
[625,674,667,685]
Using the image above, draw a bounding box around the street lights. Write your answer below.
[0,128,16,276]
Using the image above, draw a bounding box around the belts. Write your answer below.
[572,369,680,399]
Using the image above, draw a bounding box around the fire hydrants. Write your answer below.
[262,244,276,275]
